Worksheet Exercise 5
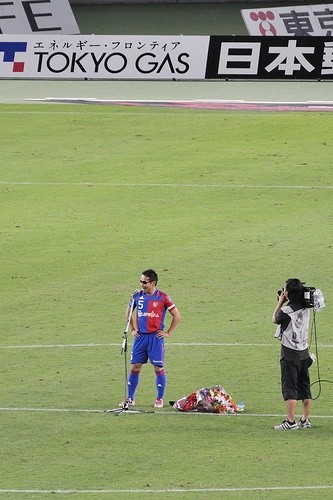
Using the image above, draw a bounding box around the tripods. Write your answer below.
[104,298,155,416]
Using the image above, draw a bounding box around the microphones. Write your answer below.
[130,288,140,297]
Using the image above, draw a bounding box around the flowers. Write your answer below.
[169,385,246,414]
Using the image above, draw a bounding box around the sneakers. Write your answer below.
[273,418,299,430]
[154,398,163,407]
[119,398,136,406]
[297,416,311,428]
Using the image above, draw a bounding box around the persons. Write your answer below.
[273,277,313,430]
[118,269,182,410]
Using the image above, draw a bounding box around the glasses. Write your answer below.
[140,280,156,284]
